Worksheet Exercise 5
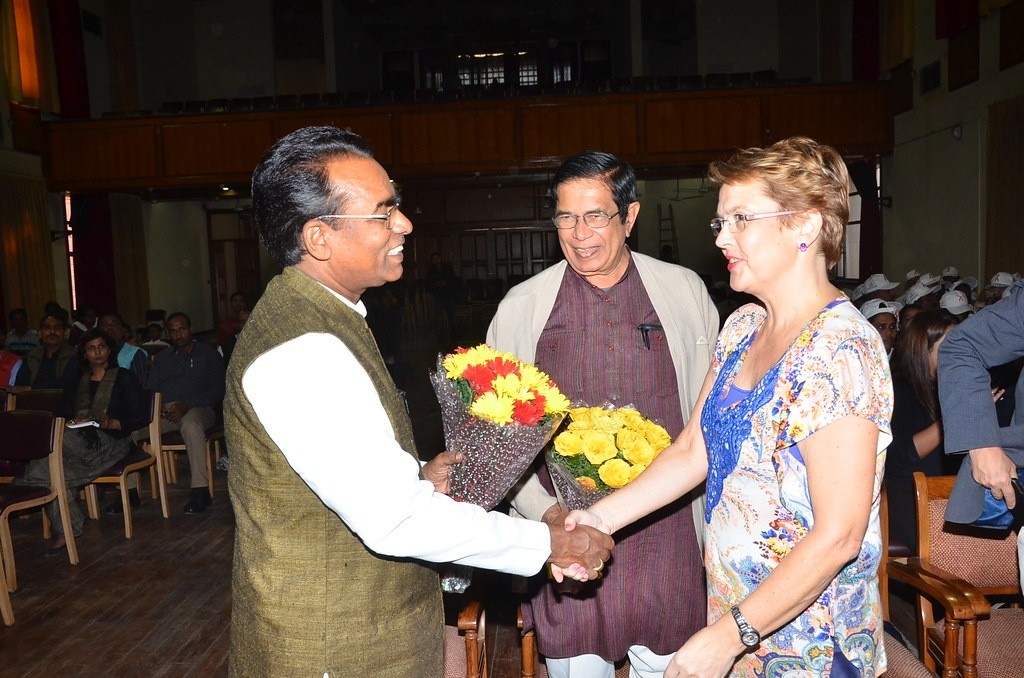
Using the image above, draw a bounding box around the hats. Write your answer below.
[850,267,1023,321]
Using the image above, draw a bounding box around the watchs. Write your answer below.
[730,605,760,650]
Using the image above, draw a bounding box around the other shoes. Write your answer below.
[104,490,141,515]
[44,544,66,557]
[183,488,212,515]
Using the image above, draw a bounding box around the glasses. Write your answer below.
[313,189,400,231]
[551,200,634,229]
[710,209,808,237]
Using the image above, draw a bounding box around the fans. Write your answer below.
[400,176,437,215]
[650,168,706,202]
[518,171,555,200]
[210,183,253,213]
[524,184,557,209]
[673,167,724,194]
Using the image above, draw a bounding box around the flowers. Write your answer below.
[439,341,675,518]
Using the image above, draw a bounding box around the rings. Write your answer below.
[593,559,604,572]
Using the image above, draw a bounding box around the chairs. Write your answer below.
[98,70,776,118]
[516,596,631,678]
[878,480,974,678]
[0,382,225,627]
[443,592,488,678]
[912,470,1024,678]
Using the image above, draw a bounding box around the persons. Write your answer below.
[485,149,720,678]
[223,127,614,678]
[835,271,1024,594]
[551,139,894,678]
[0,291,252,554]
